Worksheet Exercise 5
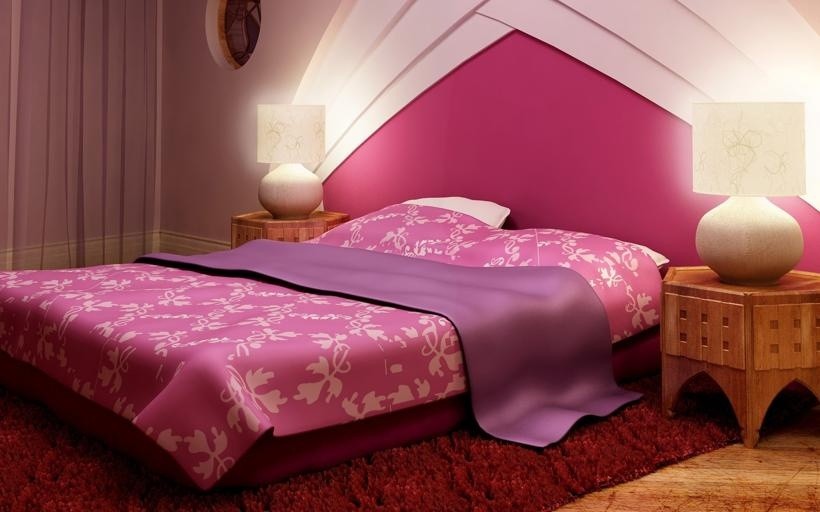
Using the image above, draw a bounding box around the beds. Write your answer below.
[0,197,675,487]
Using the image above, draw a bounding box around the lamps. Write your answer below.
[256,103,327,219]
[690,100,808,287]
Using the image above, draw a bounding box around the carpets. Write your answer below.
[0,374,815,511]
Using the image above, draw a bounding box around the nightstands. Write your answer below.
[231,211,349,249]
[660,265,819,447]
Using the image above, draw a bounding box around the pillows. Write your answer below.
[400,197,512,228]
[622,240,669,271]
